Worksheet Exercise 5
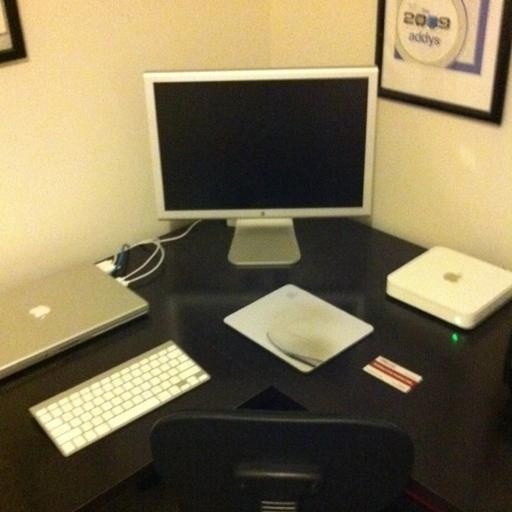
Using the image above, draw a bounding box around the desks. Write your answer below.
[0,208,512,512]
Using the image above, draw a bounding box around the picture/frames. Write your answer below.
[0,0,27,65]
[374,0,512,125]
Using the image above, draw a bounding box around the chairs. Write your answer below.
[145,409,417,512]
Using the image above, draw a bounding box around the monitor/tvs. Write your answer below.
[142,65,379,269]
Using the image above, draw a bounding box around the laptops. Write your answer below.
[0,259,150,379]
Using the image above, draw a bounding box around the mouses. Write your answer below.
[266,318,339,368]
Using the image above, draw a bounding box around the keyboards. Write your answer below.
[27,339,213,458]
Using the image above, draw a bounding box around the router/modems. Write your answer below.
[385,245,512,331]
